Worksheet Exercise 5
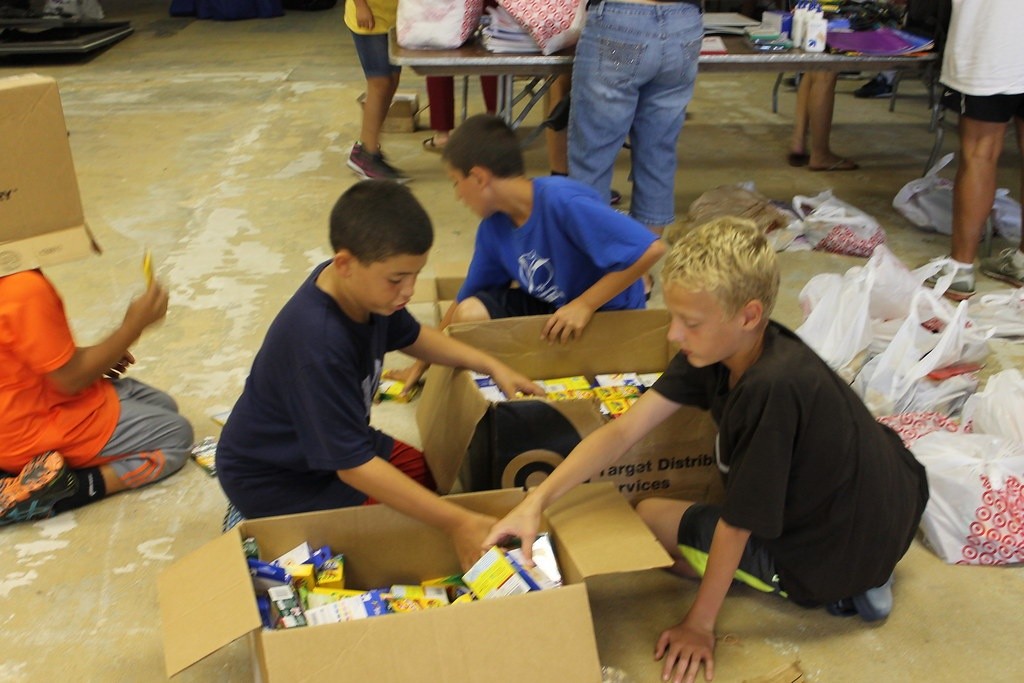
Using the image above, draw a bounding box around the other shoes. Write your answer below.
[347,140,408,184]
[981,247,1024,287]
[853,78,894,98]
[823,573,898,622]
[916,255,978,300]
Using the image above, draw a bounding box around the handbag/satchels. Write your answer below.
[791,244,1023,566]
[395,0,482,50]
[891,152,1021,242]
[793,188,885,257]
[493,0,587,56]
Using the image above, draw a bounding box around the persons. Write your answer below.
[480,215,930,683]
[915,0,1024,300]
[0,270,194,527]
[344,0,415,185]
[855,70,898,98]
[384,114,667,399]
[421,0,704,237]
[788,71,861,171]
[215,177,546,575]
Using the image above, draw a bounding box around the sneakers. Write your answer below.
[1,450,79,529]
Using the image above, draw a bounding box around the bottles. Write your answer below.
[792,2,828,54]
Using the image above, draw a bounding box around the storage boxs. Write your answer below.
[801,19,828,53]
[355,92,419,135]
[153,275,725,683]
[765,10,792,40]
[0,71,104,281]
[792,9,823,49]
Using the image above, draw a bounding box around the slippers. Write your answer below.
[787,150,859,172]
[422,135,447,151]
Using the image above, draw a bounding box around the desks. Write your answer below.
[384,23,993,258]
